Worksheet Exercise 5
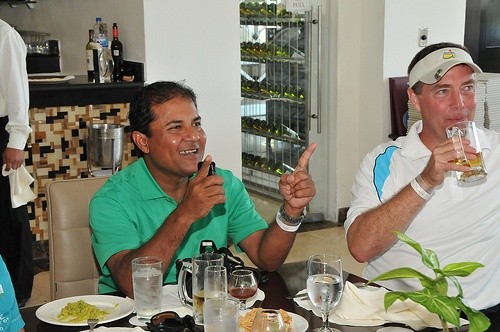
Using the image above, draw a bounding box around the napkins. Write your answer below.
[335,280,470,331]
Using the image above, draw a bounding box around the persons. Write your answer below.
[0,18,37,332]
[89,82,318,294]
[344,41,500,332]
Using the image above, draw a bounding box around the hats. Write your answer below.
[408,47,482,88]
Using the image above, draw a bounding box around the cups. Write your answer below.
[131,256,163,322]
[202,299,239,332]
[445,122,487,187]
[204,266,228,302]
[178,253,224,326]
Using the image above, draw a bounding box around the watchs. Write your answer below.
[278,200,308,222]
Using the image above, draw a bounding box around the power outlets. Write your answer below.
[418,27,428,47]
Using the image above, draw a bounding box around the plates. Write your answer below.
[26,76,76,84]
[239,287,315,332]
[35,294,146,332]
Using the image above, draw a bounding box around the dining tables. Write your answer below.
[19,259,469,332]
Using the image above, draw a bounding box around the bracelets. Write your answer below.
[275,209,301,233]
[410,175,435,201]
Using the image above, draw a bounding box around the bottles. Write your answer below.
[111,23,123,84]
[241,115,301,144]
[240,1,307,28]
[240,41,305,66]
[86,18,113,84]
[242,151,294,177]
[241,72,305,107]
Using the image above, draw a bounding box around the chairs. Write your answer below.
[44,176,110,301]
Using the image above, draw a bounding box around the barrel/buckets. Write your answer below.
[85,123,125,177]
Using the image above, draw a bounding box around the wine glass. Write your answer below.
[13,25,51,55]
[228,270,257,310]
[307,254,344,332]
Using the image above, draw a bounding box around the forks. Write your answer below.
[88,317,98,332]
